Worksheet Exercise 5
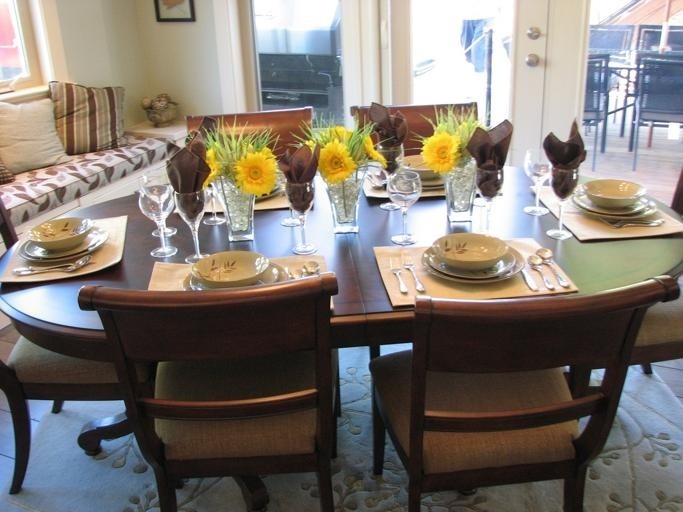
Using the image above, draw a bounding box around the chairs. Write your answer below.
[77,273,341,511]
[187,107,313,177]
[368,274,681,512]
[348,103,478,171]
[581,48,683,172]
[572,161,683,397]
[1,196,187,493]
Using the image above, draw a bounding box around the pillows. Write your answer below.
[0,163,17,184]
[49,81,130,154]
[0,97,71,173]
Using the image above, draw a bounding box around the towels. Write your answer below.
[163,116,216,221]
[276,142,321,212]
[543,118,588,200]
[466,119,512,197]
[368,101,407,170]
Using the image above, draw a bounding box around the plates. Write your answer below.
[573,190,613,271]
[420,242,526,286]
[421,179,444,191]
[183,261,288,292]
[17,228,111,262]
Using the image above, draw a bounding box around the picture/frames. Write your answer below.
[154,0,195,23]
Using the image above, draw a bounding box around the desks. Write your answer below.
[127,119,191,149]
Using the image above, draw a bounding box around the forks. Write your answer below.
[597,214,668,230]
[389,251,426,294]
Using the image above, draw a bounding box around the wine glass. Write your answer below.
[172,186,225,265]
[522,148,579,240]
[135,174,177,258]
[475,167,503,232]
[280,183,319,256]
[386,171,422,245]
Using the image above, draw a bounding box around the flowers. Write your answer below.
[189,117,282,197]
[288,115,390,185]
[410,100,488,176]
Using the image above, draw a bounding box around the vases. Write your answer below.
[213,176,259,242]
[324,179,364,234]
[442,158,477,219]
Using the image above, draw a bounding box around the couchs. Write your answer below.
[1,80,167,251]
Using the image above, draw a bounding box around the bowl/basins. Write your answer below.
[192,250,270,287]
[144,101,178,123]
[433,233,510,271]
[25,217,96,252]
[391,152,442,179]
[582,179,648,211]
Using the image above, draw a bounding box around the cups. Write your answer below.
[377,144,404,211]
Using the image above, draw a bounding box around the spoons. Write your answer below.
[527,247,571,291]
